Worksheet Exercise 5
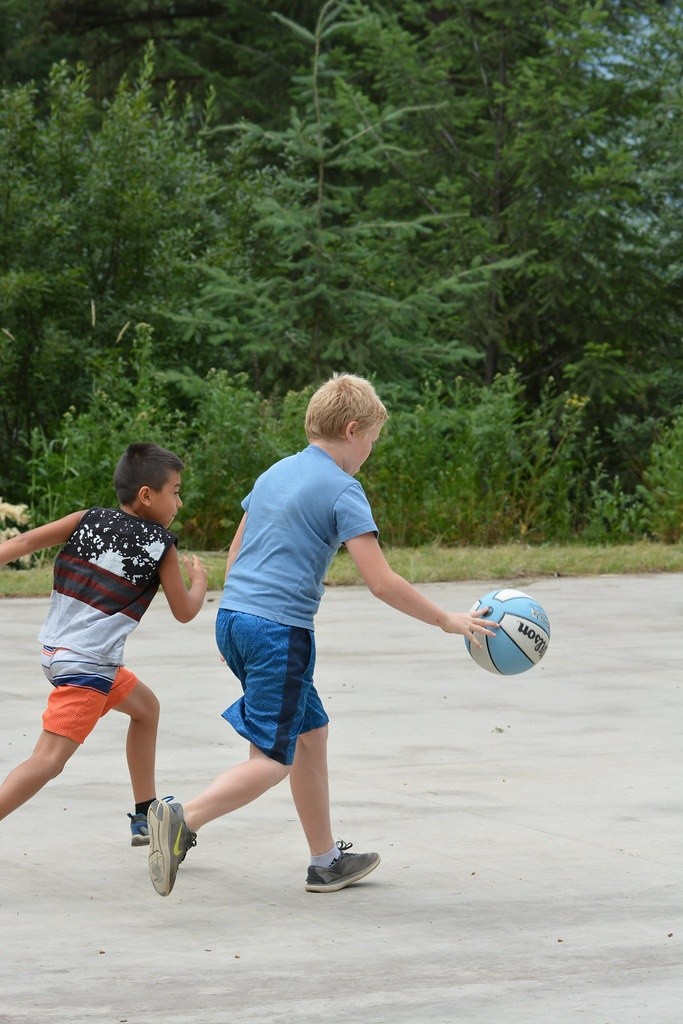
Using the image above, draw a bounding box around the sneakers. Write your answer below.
[305,840,381,893]
[147,800,197,897]
[127,796,175,846]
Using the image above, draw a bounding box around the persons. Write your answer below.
[0,441,208,845]
[149,374,500,897]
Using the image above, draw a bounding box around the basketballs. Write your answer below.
[463,586,552,678]
